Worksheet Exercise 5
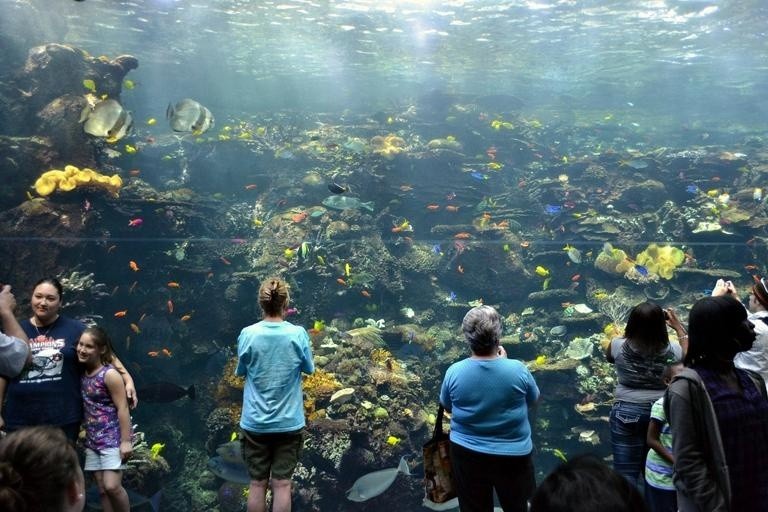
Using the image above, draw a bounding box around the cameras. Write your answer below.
[664,311,670,320]
[723,282,732,293]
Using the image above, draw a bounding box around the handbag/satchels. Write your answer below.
[423,407,459,504]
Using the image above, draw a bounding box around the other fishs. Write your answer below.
[74,76,768,462]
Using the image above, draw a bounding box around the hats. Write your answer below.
[753,277,768,309]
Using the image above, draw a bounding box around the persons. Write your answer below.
[0,277,33,378]
[608,272,768,512]
[531,452,646,512]
[0,425,86,512]
[439,304,541,512]
[78,326,130,512]
[1,276,137,442]
[233,280,314,512]
